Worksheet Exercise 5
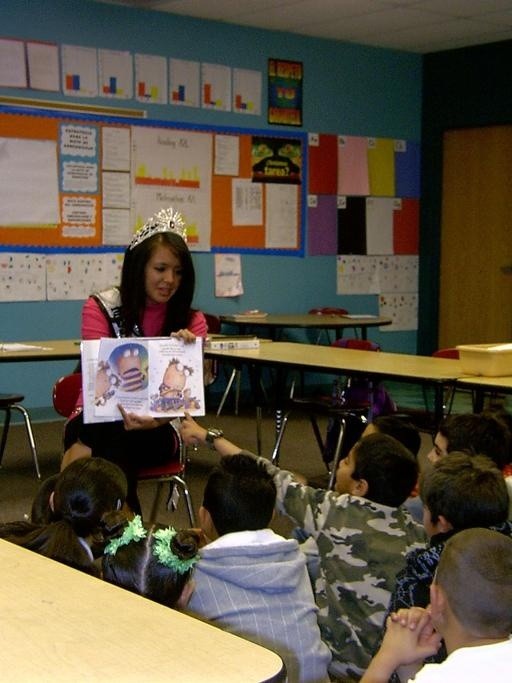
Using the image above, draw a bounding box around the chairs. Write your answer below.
[0,394,42,479]
[138,420,198,530]
[316,332,381,460]
[52,373,84,414]
[309,308,347,318]
[204,313,221,392]
[435,348,460,357]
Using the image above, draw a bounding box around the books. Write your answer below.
[79,335,205,424]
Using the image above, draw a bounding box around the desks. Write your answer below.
[0,539,288,683]
[0,340,87,365]
[457,376,512,413]
[203,341,456,486]
[227,311,392,415]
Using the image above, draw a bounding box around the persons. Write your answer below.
[53,205,212,526]
[2,411,511,683]
